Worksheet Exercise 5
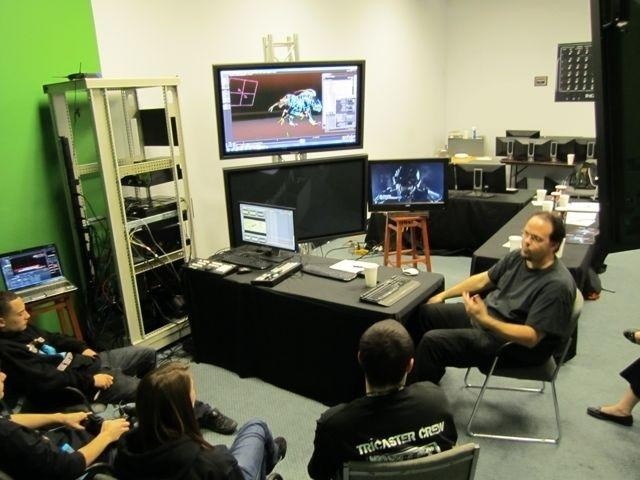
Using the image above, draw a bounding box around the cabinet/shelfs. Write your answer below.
[41,72,200,356]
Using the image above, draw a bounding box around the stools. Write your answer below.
[22,292,86,347]
[382,213,432,275]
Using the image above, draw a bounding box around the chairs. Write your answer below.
[464,288,585,446]
[0,353,110,417]
[0,421,118,480]
[339,444,480,480]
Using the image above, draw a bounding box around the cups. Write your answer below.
[363,263,378,288]
[537,189,547,204]
[552,191,560,207]
[555,185,567,195]
[567,154,575,165]
[509,235,523,252]
[542,200,553,213]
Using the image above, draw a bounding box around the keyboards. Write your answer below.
[358,274,422,307]
[222,253,273,272]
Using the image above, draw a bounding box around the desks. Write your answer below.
[501,159,586,194]
[366,188,536,259]
[188,248,445,408]
[470,192,598,297]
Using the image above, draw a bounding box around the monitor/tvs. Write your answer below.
[212,61,366,159]
[237,199,298,262]
[362,156,448,212]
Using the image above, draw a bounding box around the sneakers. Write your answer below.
[199,408,238,435]
[267,437,286,475]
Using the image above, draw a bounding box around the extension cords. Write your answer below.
[354,248,369,256]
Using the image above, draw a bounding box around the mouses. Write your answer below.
[401,265,419,276]
[236,266,250,275]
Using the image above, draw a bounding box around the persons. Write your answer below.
[0,290,238,435]
[308,318,458,480]
[406,211,577,388]
[587,328,640,426]
[0,369,130,480]
[110,361,287,480]
[375,167,441,203]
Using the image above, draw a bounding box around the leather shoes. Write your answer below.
[588,407,633,426]
[623,329,640,344]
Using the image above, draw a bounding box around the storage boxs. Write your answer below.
[448,136,485,156]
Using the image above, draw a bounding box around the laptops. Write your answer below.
[0,242,78,306]
